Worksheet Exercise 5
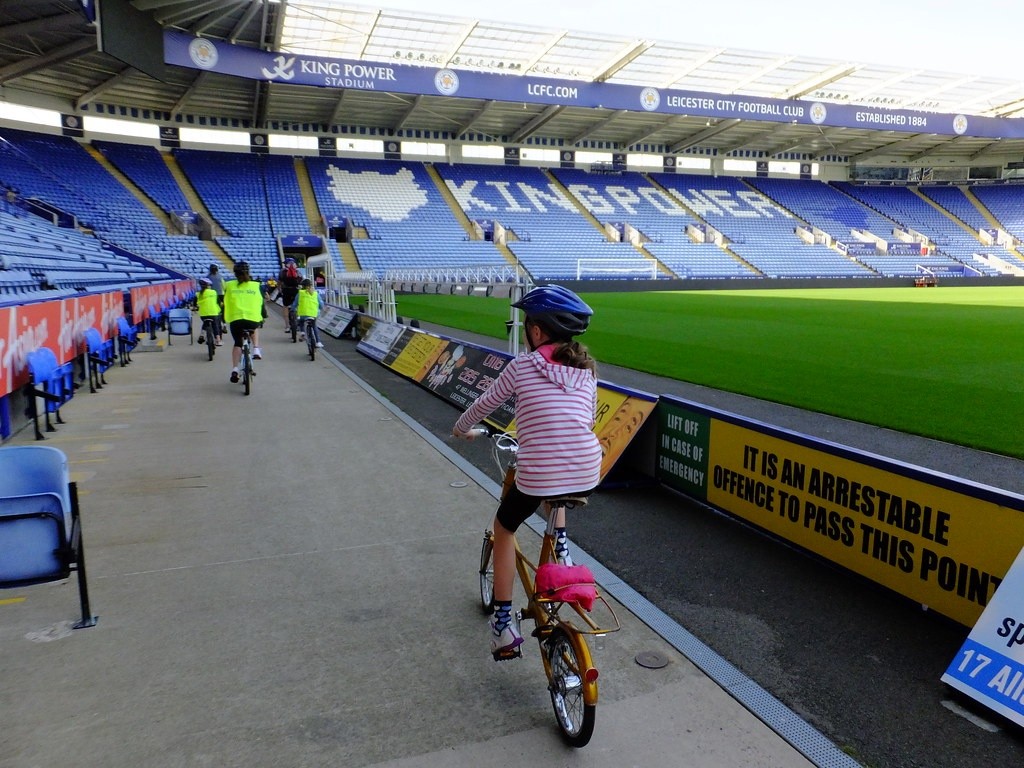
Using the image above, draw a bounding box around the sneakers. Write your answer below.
[229,367,240,384]
[300,332,305,337]
[488,613,525,656]
[551,554,575,568]
[315,342,324,348]
[253,348,262,360]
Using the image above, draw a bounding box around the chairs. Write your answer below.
[0,130,1024,443]
[0,446,100,630]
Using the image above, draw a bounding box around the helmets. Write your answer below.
[208,264,219,272]
[301,279,313,287]
[281,257,294,266]
[510,284,594,353]
[198,277,212,288]
[232,262,250,272]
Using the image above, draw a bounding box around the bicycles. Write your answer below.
[198,315,222,361]
[286,305,322,361]
[218,315,265,395]
[443,423,618,747]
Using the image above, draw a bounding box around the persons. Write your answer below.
[195,265,228,347]
[452,284,602,655]
[276,258,325,348]
[222,262,269,383]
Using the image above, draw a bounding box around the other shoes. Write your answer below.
[285,327,290,333]
[198,334,206,343]
[216,342,223,347]
[223,324,227,334]
